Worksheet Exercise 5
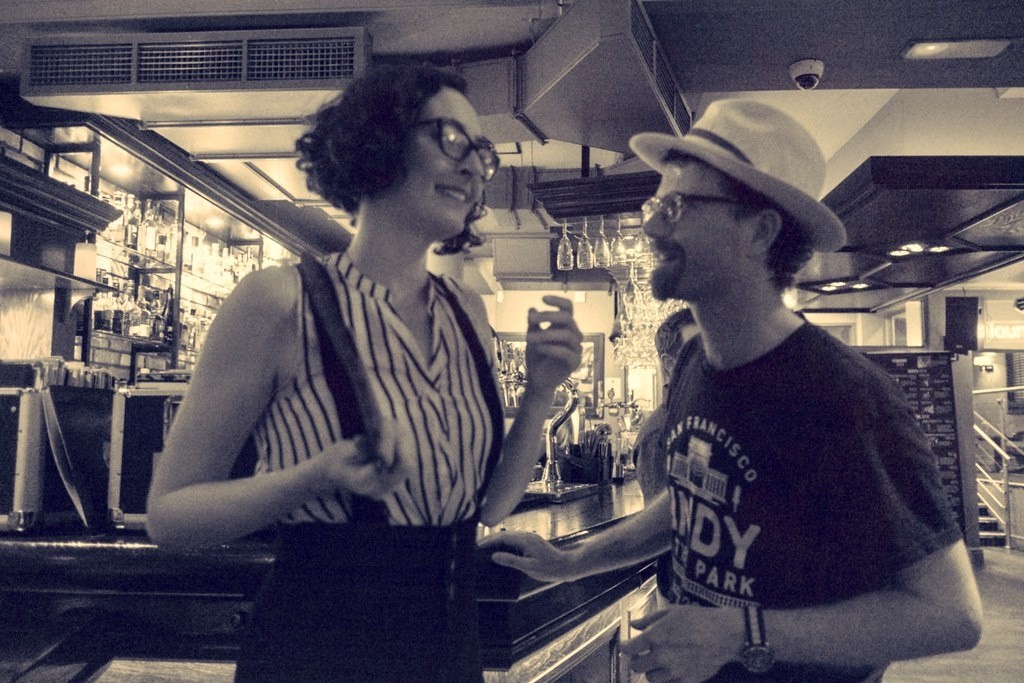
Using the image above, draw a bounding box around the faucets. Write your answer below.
[498,370,580,490]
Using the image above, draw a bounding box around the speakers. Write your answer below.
[945,296,986,350]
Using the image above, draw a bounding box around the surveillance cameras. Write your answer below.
[788,59,824,90]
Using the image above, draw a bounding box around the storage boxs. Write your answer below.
[0,388,41,531]
[107,382,189,530]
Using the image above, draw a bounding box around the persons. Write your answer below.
[478,96,983,683]
[146,65,584,683]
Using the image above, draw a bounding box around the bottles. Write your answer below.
[95,267,173,344]
[85,177,170,263]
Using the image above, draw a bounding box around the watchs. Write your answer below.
[738,605,774,673]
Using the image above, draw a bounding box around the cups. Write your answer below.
[612,463,624,482]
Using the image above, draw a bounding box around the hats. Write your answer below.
[629,98,848,253]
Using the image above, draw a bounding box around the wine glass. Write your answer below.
[594,215,610,268]
[612,212,686,370]
[557,218,573,271]
[577,217,594,269]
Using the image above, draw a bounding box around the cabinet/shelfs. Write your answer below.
[0,152,124,396]
[44,142,281,383]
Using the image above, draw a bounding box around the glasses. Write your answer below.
[412,117,500,182]
[641,193,755,225]
